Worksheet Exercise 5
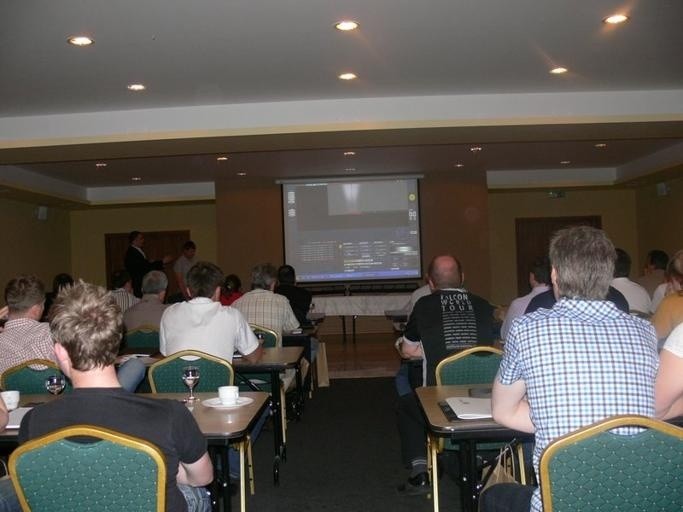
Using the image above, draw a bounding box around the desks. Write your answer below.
[305,312,325,332]
[113,347,306,486]
[281,330,312,417]
[415,384,536,512]
[0,391,270,511]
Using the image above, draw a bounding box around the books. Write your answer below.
[437,386,496,424]
[122,347,159,356]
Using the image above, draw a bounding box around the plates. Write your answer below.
[201,397,253,411]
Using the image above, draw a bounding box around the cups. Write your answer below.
[219,387,238,404]
[0,391,19,410]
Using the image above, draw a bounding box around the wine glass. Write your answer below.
[46,376,66,400]
[183,368,200,400]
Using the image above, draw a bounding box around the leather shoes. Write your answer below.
[393,472,430,499]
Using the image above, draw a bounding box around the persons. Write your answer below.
[174,241,199,303]
[230,264,308,391]
[652,319,683,423]
[42,270,73,320]
[646,249,683,352]
[269,265,320,363]
[104,271,141,313]
[499,259,553,341]
[0,273,144,396]
[611,248,651,316]
[218,274,242,305]
[122,270,172,331]
[632,249,668,299]
[478,225,661,512]
[19,279,215,512]
[396,255,495,499]
[647,271,674,312]
[391,270,432,400]
[158,261,272,495]
[124,228,174,296]
[524,286,629,317]
[0,392,21,512]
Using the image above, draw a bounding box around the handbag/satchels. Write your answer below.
[479,444,519,497]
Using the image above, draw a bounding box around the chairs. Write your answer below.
[424,344,516,512]
[383,308,409,325]
[7,425,171,511]
[146,350,233,512]
[233,322,286,444]
[119,324,161,356]
[541,414,683,512]
[1,360,76,396]
